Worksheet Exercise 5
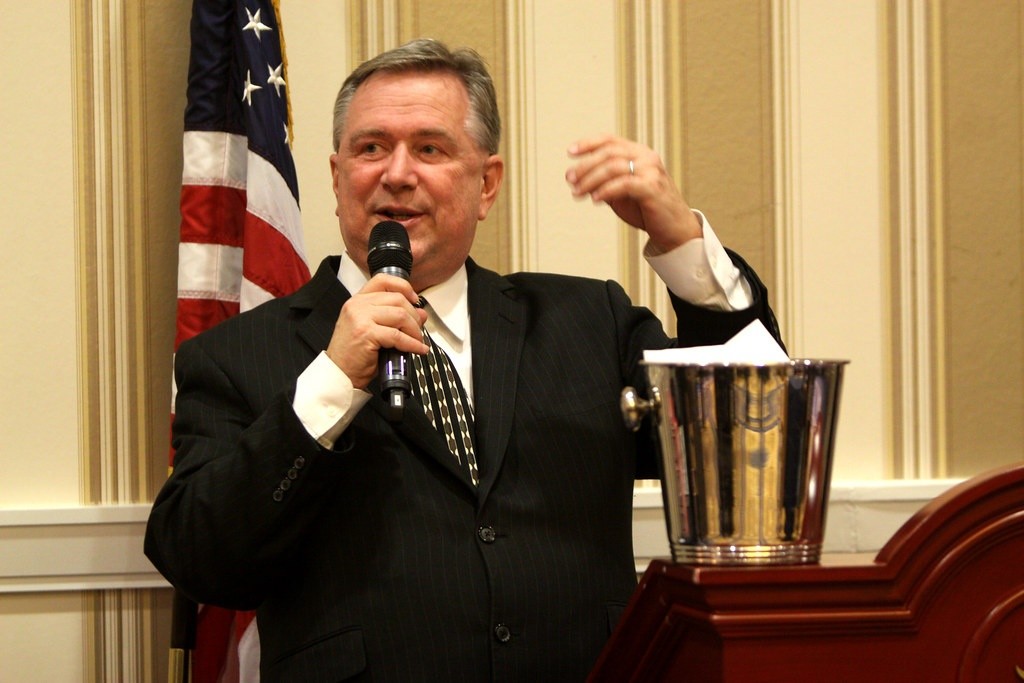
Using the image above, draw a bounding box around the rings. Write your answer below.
[626,158,636,173]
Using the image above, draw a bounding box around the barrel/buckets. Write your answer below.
[620,359,850,563]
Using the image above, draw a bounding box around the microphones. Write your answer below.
[366,219,414,418]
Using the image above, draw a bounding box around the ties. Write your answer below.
[409,296,481,490]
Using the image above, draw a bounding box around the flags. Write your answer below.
[165,1,313,681]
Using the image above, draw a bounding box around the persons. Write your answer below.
[145,37,793,682]
